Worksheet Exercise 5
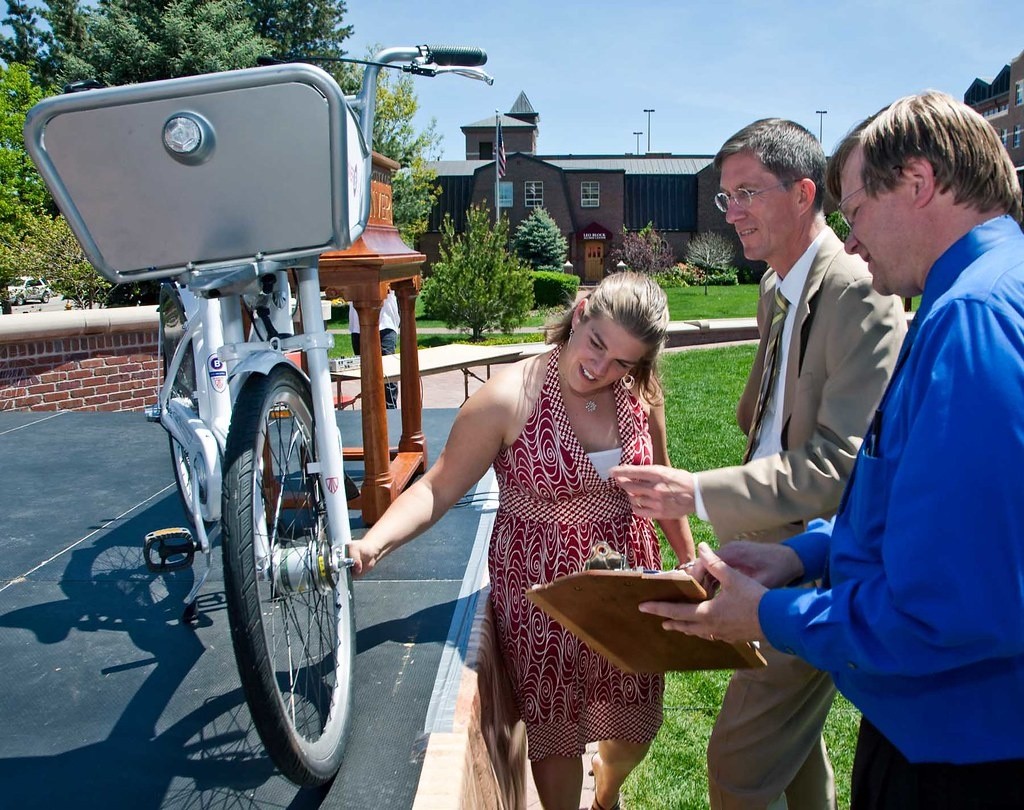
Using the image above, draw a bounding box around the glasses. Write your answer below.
[715,179,804,212]
[836,165,902,229]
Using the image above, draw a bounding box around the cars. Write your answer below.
[1,274,50,306]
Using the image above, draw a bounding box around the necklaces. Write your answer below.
[572,390,601,413]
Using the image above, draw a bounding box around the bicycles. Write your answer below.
[19,44,495,791]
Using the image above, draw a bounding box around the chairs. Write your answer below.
[284,295,355,411]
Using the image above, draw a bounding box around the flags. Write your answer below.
[493,118,506,179]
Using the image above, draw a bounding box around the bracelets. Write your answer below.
[675,562,694,571]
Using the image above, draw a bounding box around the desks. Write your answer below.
[330,343,523,408]
[260,150,430,528]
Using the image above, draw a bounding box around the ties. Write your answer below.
[745,288,791,466]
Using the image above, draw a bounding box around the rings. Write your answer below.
[636,497,643,508]
[710,634,716,641]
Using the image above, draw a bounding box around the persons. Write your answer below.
[349,290,400,409]
[609,118,909,810]
[638,91,1024,810]
[349,272,697,810]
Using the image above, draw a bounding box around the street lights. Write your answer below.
[816,110,827,145]
[644,109,656,152]
[633,132,643,155]
